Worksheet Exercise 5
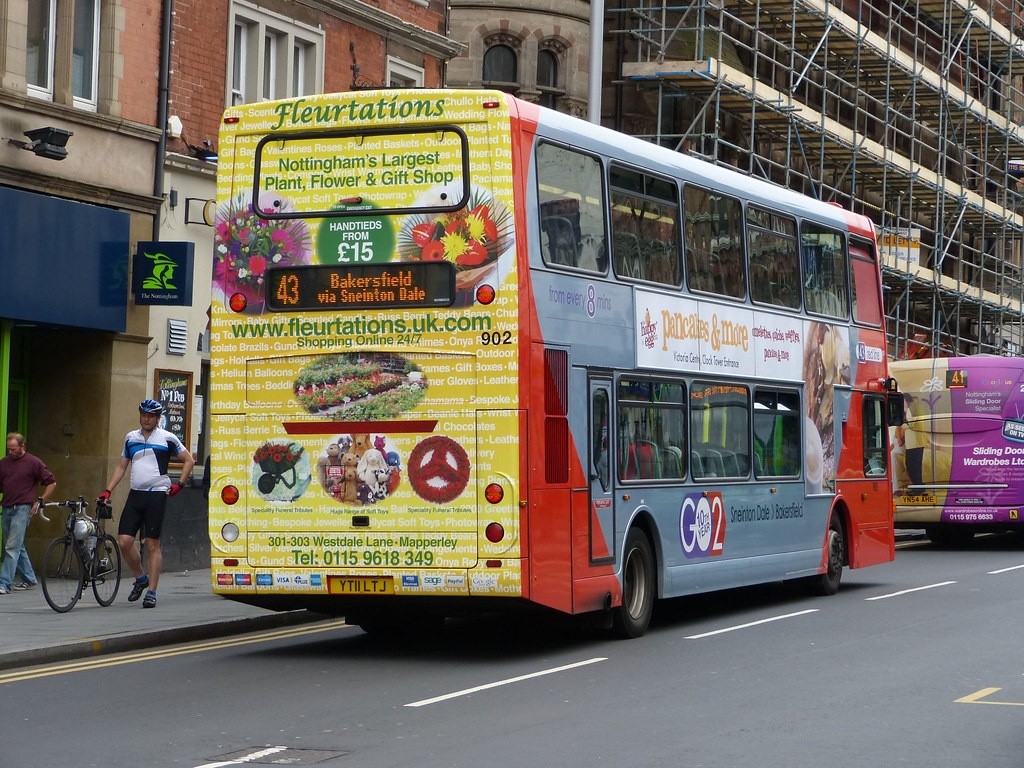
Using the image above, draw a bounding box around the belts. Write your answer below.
[3,503,31,509]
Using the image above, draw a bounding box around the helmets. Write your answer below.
[139,399,162,414]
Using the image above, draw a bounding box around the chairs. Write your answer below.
[543,214,792,306]
[619,435,762,481]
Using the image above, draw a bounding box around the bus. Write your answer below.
[874,355,1024,549]
[210,87,906,650]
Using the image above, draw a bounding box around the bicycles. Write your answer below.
[33,495,123,613]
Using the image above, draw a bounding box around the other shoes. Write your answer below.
[906,484,926,496]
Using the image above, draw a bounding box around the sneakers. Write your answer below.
[128,576,149,602]
[142,590,157,608]
[0,588,8,594]
[14,581,37,590]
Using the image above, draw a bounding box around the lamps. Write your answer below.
[5,123,74,159]
[189,145,218,160]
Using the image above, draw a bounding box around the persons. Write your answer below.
[597,431,654,488]
[902,393,926,496]
[93,399,195,608]
[0,432,56,594]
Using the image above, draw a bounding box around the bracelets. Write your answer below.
[176,481,185,488]
